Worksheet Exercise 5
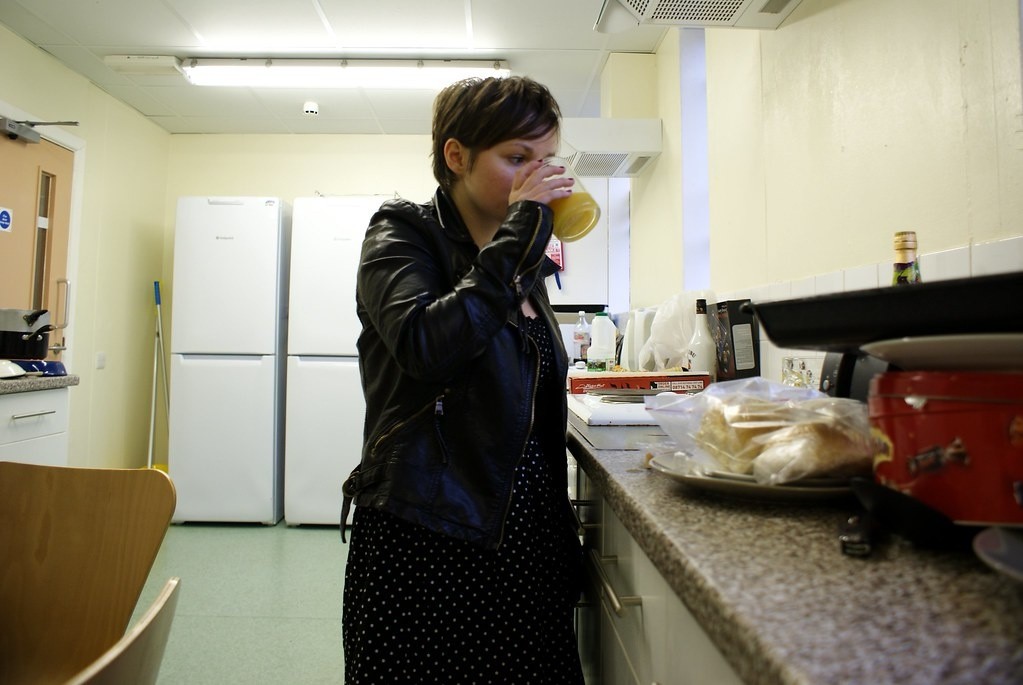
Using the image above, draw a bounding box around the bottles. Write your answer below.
[891,231,923,286]
[586,312,617,372]
[679,299,719,384]
[572,311,590,367]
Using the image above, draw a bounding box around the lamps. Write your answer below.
[182,57,511,91]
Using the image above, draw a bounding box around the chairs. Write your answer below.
[0,457,182,685]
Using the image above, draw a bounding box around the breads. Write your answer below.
[692,394,883,483]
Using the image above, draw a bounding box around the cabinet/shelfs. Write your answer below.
[0,388,68,469]
[568,457,741,685]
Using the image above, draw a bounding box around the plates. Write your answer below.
[648,450,853,496]
[859,333,1023,370]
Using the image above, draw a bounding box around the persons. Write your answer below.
[342,75,584,685]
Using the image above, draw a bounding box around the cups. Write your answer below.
[527,156,601,243]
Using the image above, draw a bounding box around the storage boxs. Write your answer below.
[567,373,709,395]
